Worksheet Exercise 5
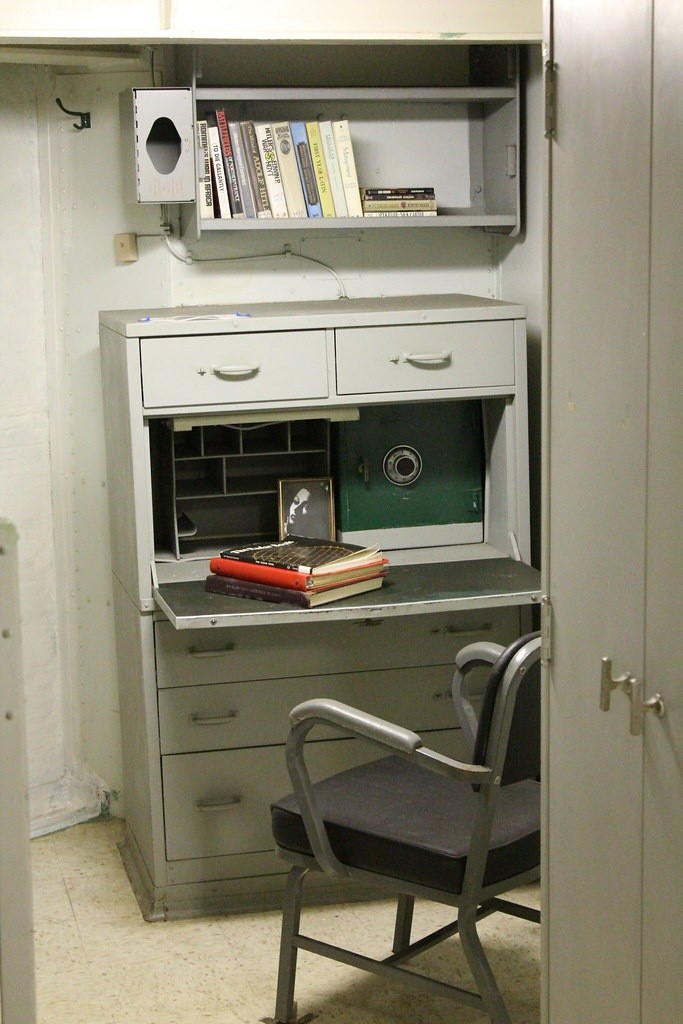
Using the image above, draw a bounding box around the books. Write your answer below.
[196,105,438,218]
[204,537,390,609]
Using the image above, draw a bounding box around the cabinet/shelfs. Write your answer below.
[110,573,544,922]
[0,516,35,1024]
[98,302,330,611]
[326,293,532,567]
[133,45,523,242]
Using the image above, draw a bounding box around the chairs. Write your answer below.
[269,630,542,1024]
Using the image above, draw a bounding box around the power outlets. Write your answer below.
[115,233,137,263]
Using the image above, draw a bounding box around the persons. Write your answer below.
[282,479,331,543]
